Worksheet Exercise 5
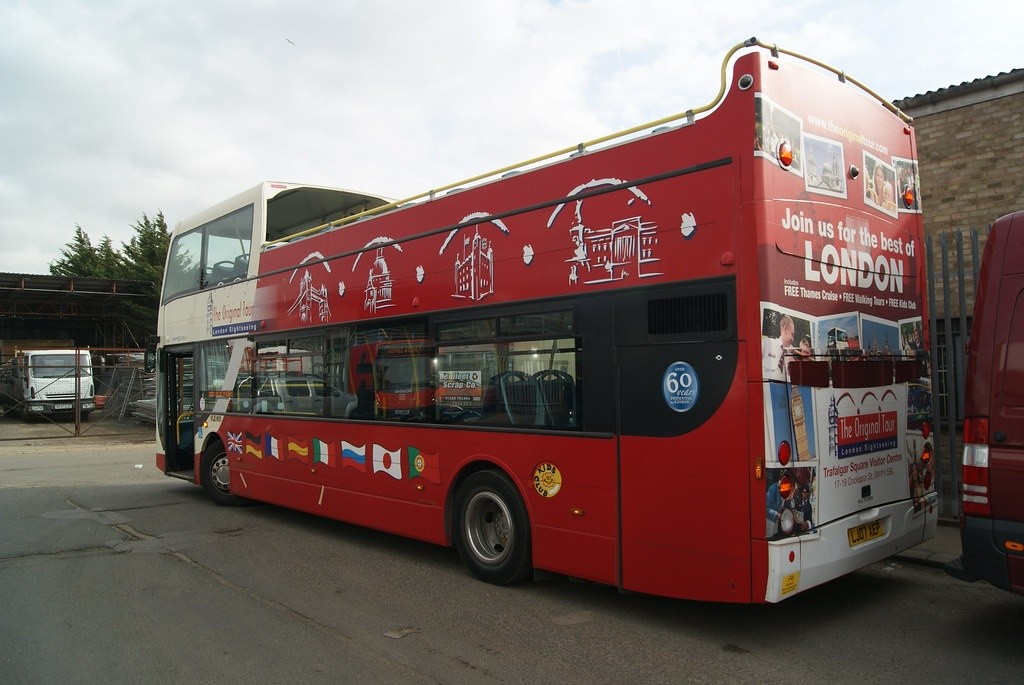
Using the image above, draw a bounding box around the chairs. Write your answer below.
[191,267,212,288]
[500,371,536,426]
[209,260,235,284]
[451,410,481,425]
[232,254,249,279]
[534,369,575,426]
[440,404,464,424]
[481,370,538,427]
[537,371,570,427]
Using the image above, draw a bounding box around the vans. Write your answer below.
[941,210,1024,600]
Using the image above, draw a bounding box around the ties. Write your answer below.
[777,346,787,370]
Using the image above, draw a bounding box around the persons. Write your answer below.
[896,166,920,210]
[910,442,935,513]
[766,470,812,541]
[870,162,890,206]
[908,390,933,419]
[903,328,922,359]
[761,314,816,382]
[754,103,798,165]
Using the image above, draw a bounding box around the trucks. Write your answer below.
[0,347,96,423]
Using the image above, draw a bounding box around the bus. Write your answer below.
[145,38,942,605]
